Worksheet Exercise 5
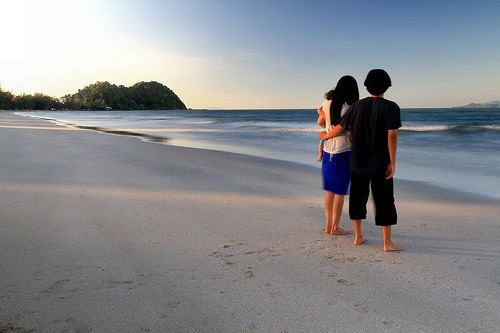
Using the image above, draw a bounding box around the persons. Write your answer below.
[316,74,359,236]
[316,90,335,162]
[320,68,405,252]
